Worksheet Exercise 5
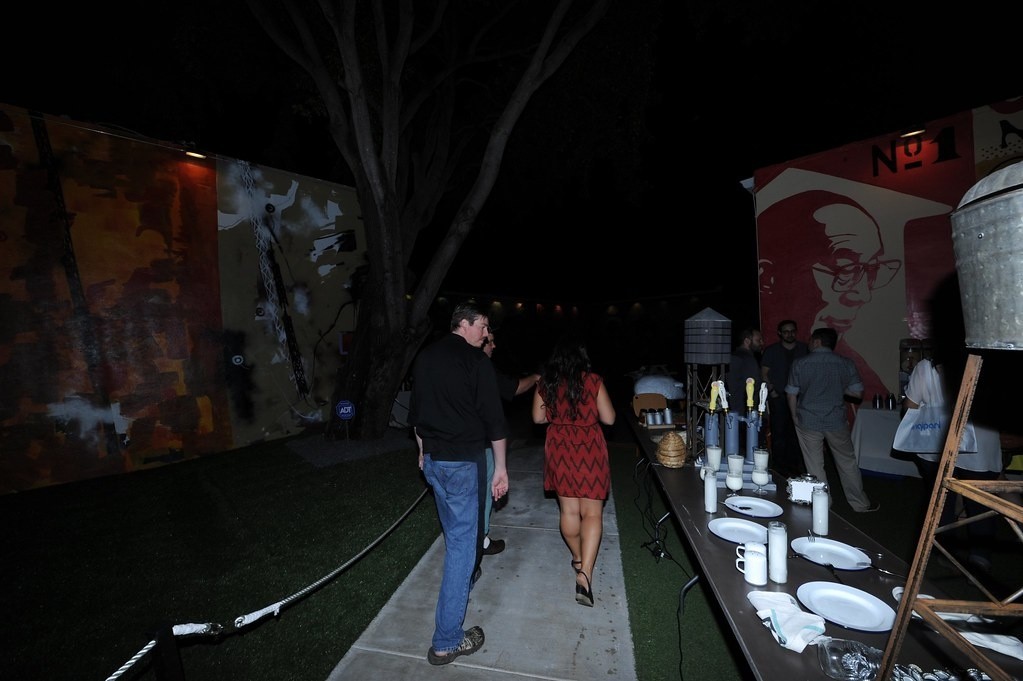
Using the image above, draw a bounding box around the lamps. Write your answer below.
[899,125,925,139]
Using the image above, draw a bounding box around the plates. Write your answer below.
[708,518,769,544]
[725,497,783,517]
[797,581,896,632]
[791,537,872,569]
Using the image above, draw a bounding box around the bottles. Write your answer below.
[640,408,672,427]
[818,639,884,681]
[886,393,896,410]
[873,394,883,410]
[768,522,787,584]
[813,487,828,535]
[705,469,717,513]
[899,338,932,375]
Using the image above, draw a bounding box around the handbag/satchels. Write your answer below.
[892,361,978,453]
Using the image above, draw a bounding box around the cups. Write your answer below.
[728,455,744,474]
[754,447,769,470]
[707,446,722,471]
[736,543,767,586]
[700,463,717,480]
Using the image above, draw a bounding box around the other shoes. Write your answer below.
[868,503,881,512]
[571,560,582,576]
[428,626,485,665]
[575,571,594,606]
[482,536,505,555]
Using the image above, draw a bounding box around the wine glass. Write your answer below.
[726,475,743,497]
[752,470,769,495]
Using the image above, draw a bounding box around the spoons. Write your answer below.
[718,501,752,510]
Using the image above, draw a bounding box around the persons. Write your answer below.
[405,302,509,666]
[479,326,541,556]
[759,319,809,479]
[729,326,774,459]
[899,337,1003,586]
[532,335,616,607]
[784,328,880,512]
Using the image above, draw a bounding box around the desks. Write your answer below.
[622,400,1023,681]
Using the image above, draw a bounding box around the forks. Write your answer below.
[808,528,815,543]
[824,563,843,584]
[857,563,907,579]
[787,553,810,559]
